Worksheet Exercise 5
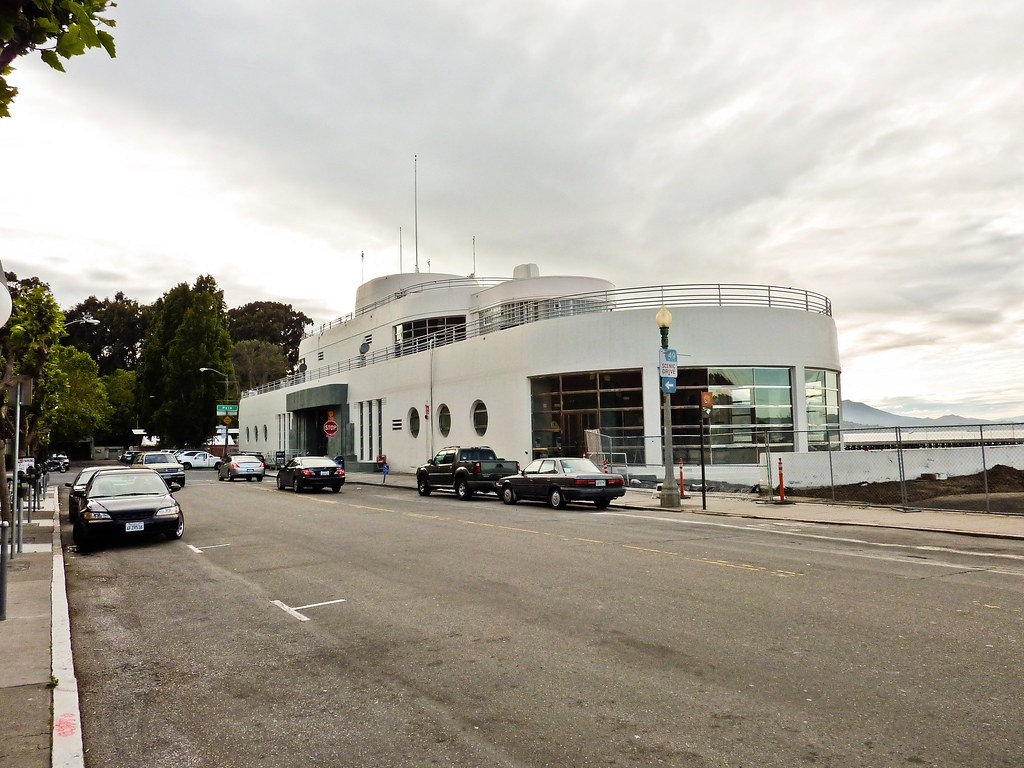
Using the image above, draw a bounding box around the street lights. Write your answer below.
[655,302,684,508]
[136,395,156,446]
[199,368,229,458]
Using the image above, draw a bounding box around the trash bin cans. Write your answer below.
[335,456,344,470]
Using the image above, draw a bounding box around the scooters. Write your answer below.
[46,460,67,473]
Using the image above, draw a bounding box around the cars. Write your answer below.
[132,450,185,488]
[217,452,266,482]
[64,465,141,523]
[73,468,184,553]
[46,450,71,470]
[276,456,346,492]
[495,457,628,511]
[117,448,224,470]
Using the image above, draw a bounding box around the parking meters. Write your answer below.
[16,463,46,553]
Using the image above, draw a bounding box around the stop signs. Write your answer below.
[323,421,339,436]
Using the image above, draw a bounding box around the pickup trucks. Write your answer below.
[415,446,521,501]
